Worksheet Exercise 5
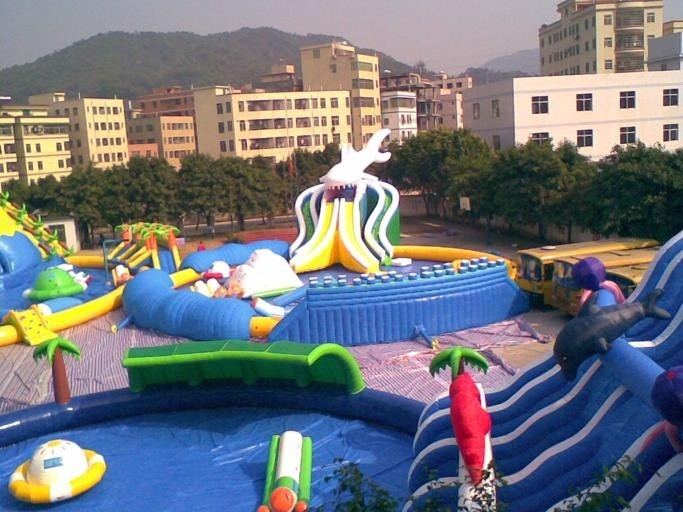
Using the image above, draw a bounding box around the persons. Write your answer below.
[196,240,206,251]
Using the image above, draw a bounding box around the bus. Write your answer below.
[506,233,660,313]
[545,250,660,317]
[595,262,655,301]
